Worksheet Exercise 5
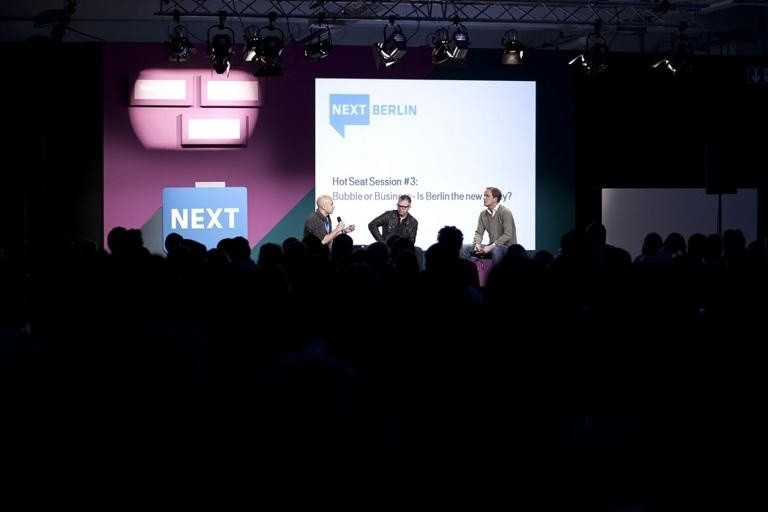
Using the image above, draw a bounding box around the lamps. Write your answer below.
[159,1,703,77]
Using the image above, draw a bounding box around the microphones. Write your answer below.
[337,217,345,234]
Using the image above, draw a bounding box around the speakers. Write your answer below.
[705,151,737,193]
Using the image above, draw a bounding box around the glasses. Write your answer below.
[397,204,408,210]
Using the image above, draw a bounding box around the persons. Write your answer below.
[303,195,355,248]
[368,195,418,244]
[458,187,517,266]
